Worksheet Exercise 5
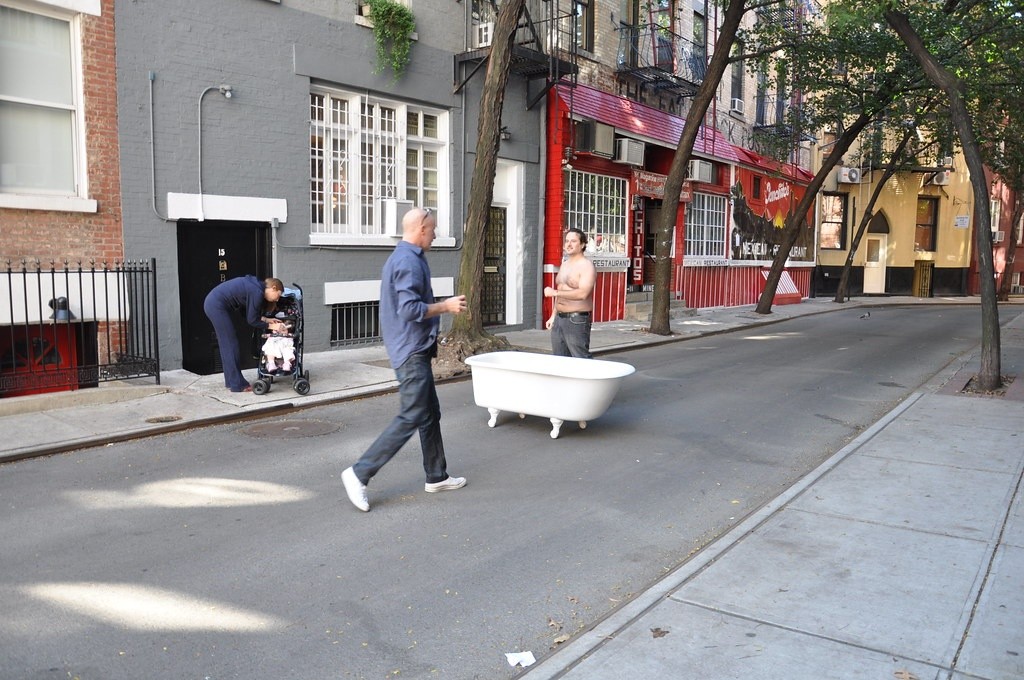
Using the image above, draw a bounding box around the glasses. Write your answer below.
[421,208,431,224]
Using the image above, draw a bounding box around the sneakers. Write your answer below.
[341,466,370,512]
[425,476,467,492]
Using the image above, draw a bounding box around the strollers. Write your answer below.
[253,282,311,395]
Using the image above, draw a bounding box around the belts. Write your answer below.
[557,311,590,318]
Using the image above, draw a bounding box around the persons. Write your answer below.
[340,210,466,510]
[261,322,296,373]
[204,276,285,390]
[543,229,597,358]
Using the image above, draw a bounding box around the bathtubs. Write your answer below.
[463,350,636,439]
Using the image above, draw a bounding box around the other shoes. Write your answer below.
[266,363,278,372]
[241,386,254,392]
[283,362,292,371]
